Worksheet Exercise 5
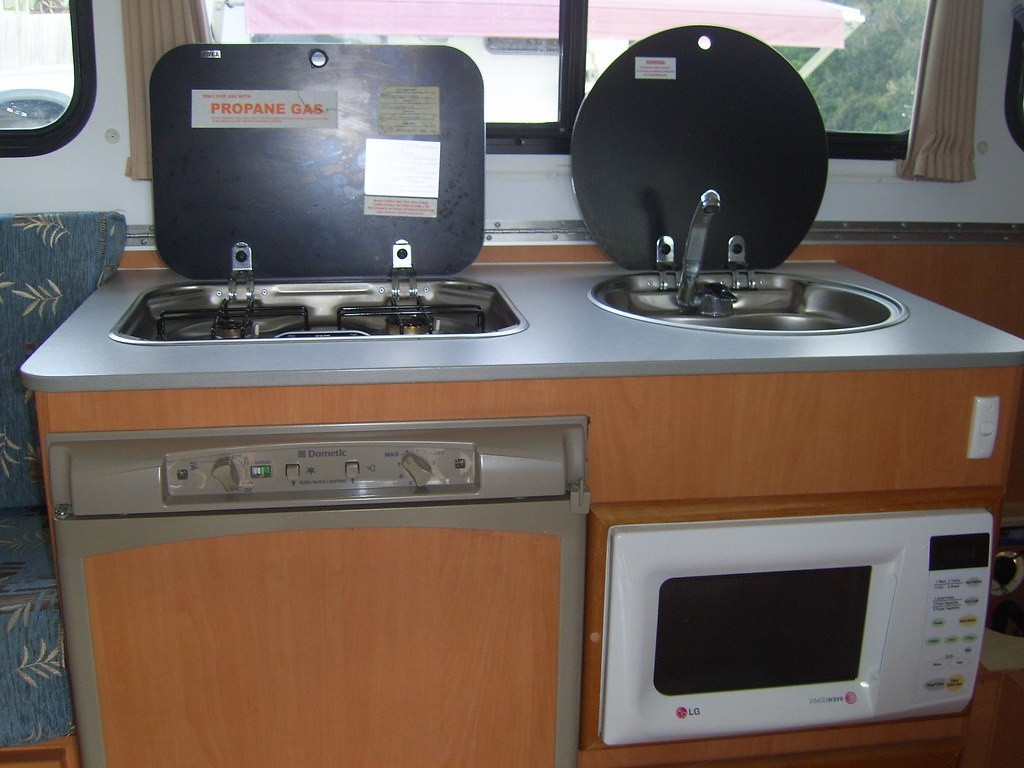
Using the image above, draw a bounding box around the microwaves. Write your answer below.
[598,508,994,746]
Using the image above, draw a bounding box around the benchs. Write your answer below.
[0,211,126,767]
